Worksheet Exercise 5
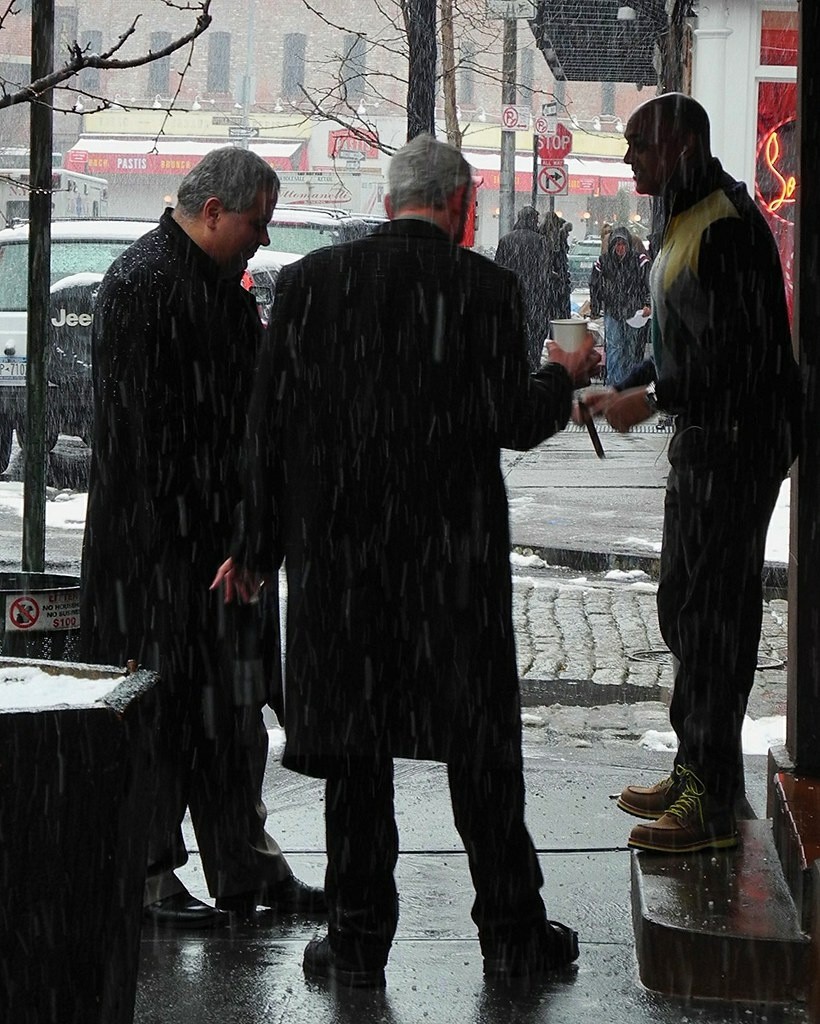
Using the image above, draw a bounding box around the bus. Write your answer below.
[1,168,108,229]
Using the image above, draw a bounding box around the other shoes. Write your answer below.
[483,920,579,974]
[302,934,387,990]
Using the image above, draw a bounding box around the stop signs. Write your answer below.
[538,122,573,161]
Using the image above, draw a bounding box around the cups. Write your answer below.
[550,320,590,353]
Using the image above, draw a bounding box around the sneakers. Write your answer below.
[616,765,739,852]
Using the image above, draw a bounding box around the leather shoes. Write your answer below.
[142,892,223,929]
[215,874,326,915]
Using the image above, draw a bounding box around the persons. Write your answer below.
[570,89,795,854]
[77,146,323,923]
[540,209,652,388]
[208,133,603,1019]
[493,206,554,372]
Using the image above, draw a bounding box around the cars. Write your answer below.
[569,240,603,292]
[0,203,398,474]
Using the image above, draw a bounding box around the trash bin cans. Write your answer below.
[0,571,82,663]
[0,652,163,1024]
